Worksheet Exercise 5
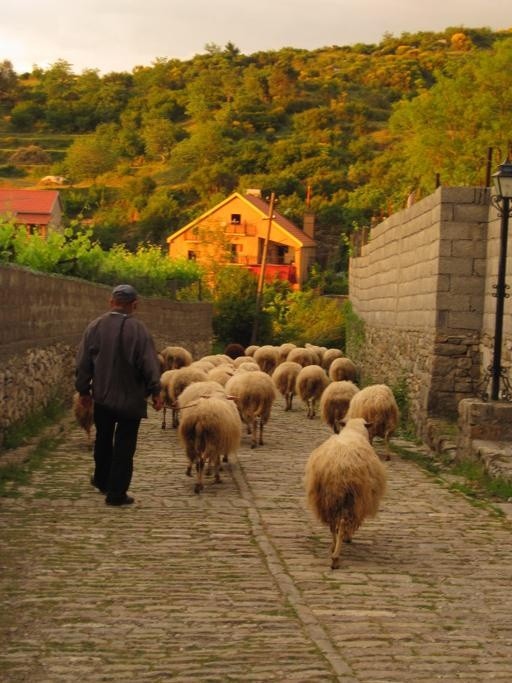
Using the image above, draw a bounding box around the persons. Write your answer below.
[75,284,165,507]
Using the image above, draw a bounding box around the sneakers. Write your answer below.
[91,475,134,505]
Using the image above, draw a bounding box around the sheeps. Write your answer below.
[301,417,390,569]
[155,342,401,496]
[72,383,96,440]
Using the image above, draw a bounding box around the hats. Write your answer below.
[112,284,141,304]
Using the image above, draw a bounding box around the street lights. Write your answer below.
[474,146,512,402]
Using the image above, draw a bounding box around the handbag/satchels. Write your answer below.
[116,362,147,417]
